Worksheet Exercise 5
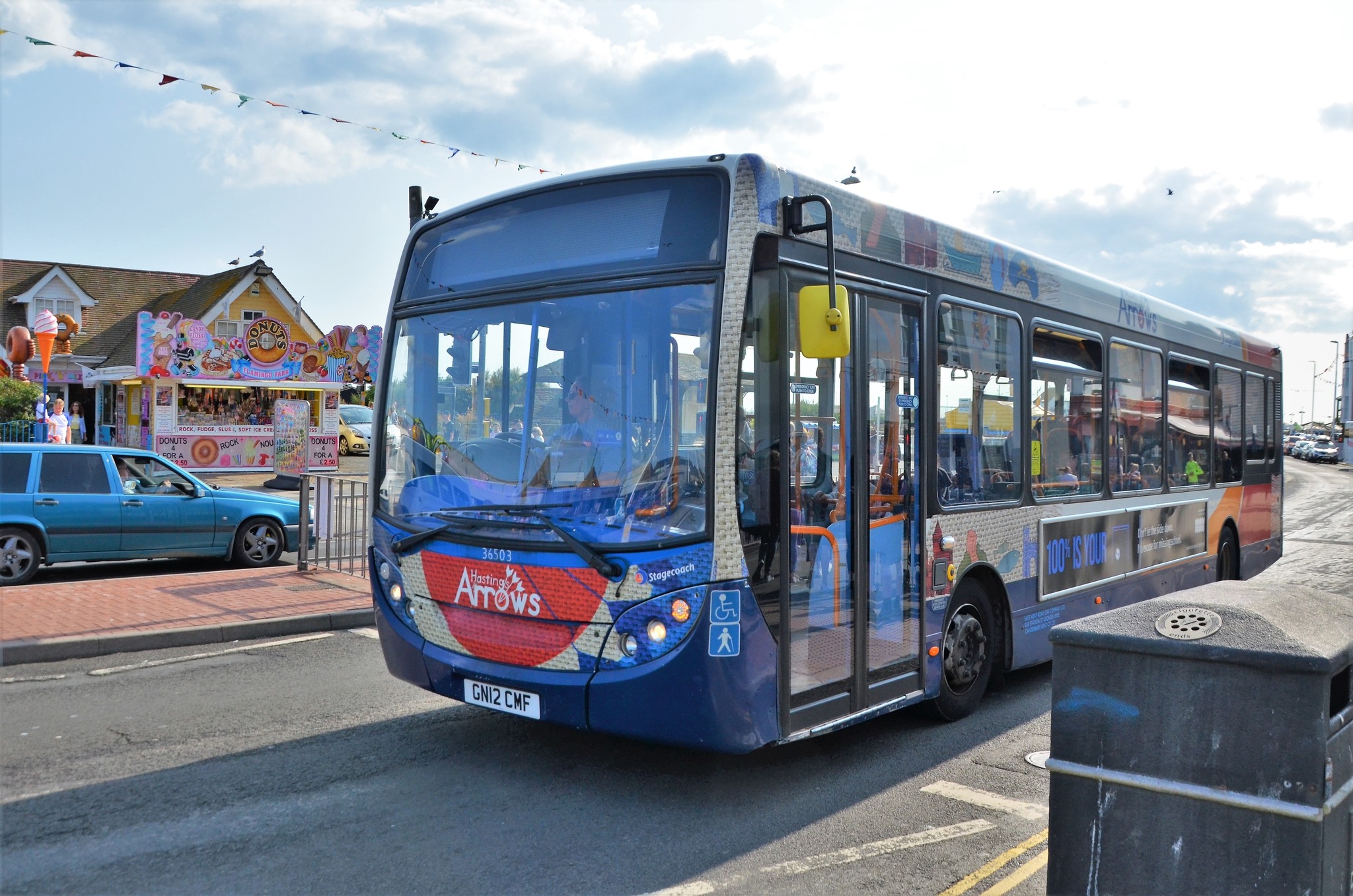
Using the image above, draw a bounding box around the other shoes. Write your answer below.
[48,434,51,441]
[51,435,60,441]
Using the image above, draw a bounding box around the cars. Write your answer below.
[339,405,401,456]
[0,442,316,586]
[1284,432,1317,460]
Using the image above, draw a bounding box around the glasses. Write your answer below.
[532,431,538,433]
[72,405,79,407]
[568,392,587,399]
[119,466,128,471]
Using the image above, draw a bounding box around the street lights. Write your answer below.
[1299,412,1305,425]
[1290,414,1294,425]
[1330,341,1338,422]
[1309,360,1316,422]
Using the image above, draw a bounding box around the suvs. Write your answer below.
[1308,434,1339,464]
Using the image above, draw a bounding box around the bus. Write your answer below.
[367,156,1283,754]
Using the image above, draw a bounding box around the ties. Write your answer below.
[575,427,582,436]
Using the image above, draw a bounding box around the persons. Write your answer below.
[439,410,459,474]
[115,459,171,495]
[739,408,953,519]
[474,512,531,536]
[545,375,625,473]
[368,401,406,428]
[485,419,544,443]
[737,450,904,620]
[935,454,1021,503]
[36,393,87,445]
[1041,451,1234,491]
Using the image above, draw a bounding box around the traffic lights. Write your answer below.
[693,336,709,369]
[444,328,471,388]
[696,411,707,435]
[696,377,707,403]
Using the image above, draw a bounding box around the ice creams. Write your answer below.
[34,309,58,373]
[348,326,370,382]
[244,438,260,465]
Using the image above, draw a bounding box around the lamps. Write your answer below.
[253,265,273,277]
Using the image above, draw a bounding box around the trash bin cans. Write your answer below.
[1045,580,1353,896]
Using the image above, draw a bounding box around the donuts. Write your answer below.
[192,438,219,465]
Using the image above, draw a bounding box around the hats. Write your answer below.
[440,410,450,415]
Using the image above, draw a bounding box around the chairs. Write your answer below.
[899,414,1224,502]
